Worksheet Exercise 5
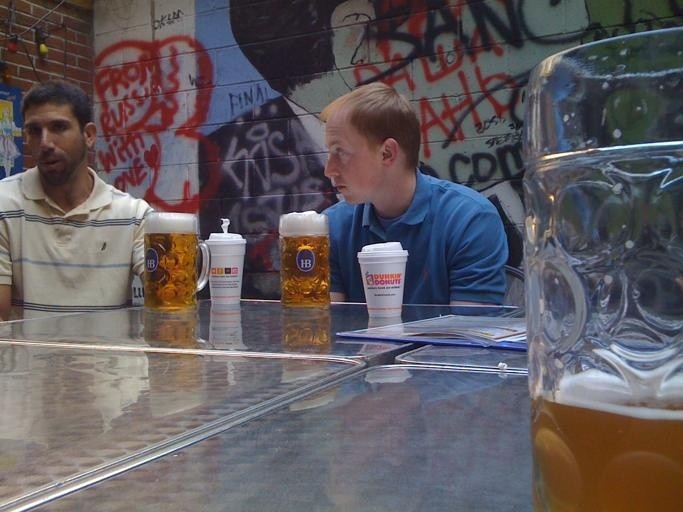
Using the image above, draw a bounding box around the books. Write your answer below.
[335,312,528,352]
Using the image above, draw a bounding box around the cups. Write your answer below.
[279,213,332,309]
[142,212,208,311]
[357,241,409,319]
[203,231,246,305]
[527,27,682,511]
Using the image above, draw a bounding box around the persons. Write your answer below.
[316,82,513,310]
[0,77,163,322]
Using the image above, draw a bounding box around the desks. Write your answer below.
[0,291,680,512]
[0,300,531,510]
